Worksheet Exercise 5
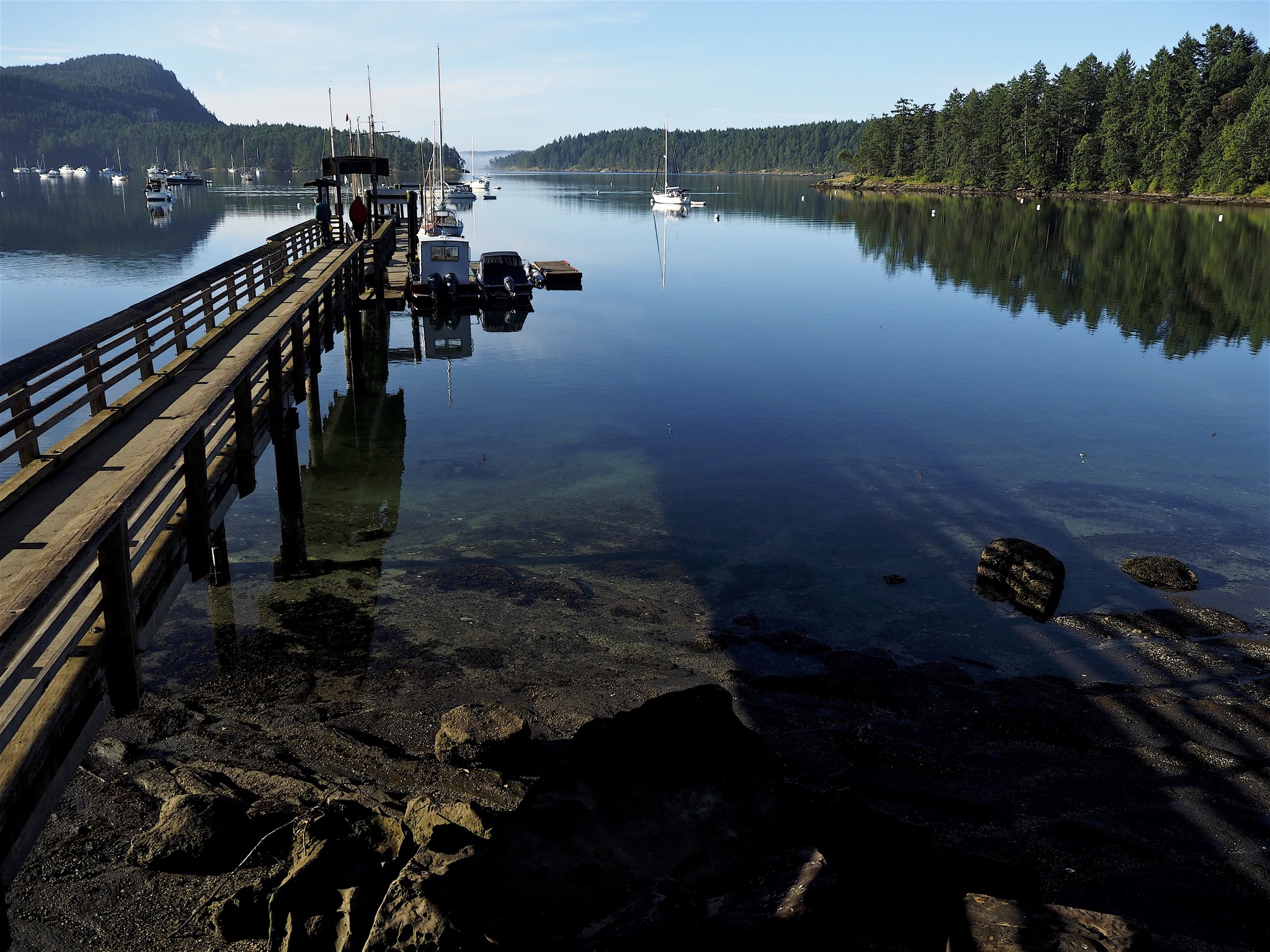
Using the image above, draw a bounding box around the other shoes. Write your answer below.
[357,239,363,242]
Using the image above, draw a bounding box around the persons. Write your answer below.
[349,196,368,241]
[313,197,332,248]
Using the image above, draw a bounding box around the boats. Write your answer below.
[146,200,174,231]
[478,309,530,334]
[422,314,473,408]
[40,164,91,178]
[167,149,205,186]
[144,145,172,200]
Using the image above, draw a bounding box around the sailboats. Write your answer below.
[255,147,263,174]
[12,154,45,174]
[98,158,117,174]
[651,114,692,205]
[99,173,111,185]
[112,180,128,214]
[111,148,129,180]
[227,154,238,173]
[652,203,691,290]
[408,43,536,302]
[240,138,254,181]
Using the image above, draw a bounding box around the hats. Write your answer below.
[355,196,361,199]
